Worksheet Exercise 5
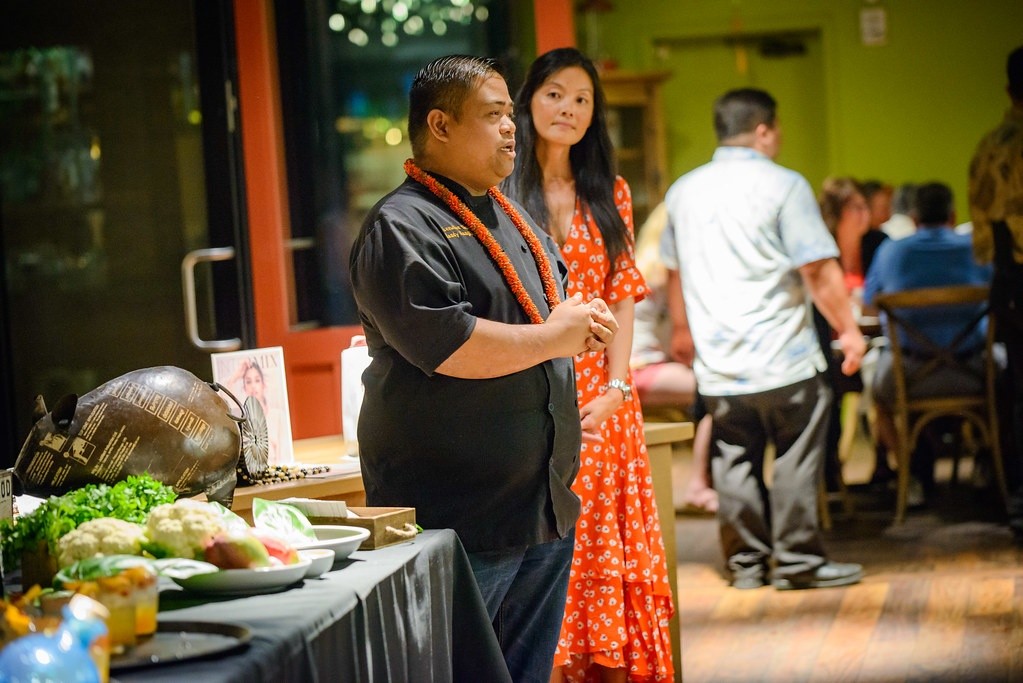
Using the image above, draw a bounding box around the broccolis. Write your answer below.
[56,499,254,571]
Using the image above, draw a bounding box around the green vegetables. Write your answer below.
[1,474,177,617]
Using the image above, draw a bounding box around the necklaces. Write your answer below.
[405,158,562,325]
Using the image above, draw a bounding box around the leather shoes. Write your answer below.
[772,557,864,591]
[732,573,771,589]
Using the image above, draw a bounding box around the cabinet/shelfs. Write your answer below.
[598,65,672,211]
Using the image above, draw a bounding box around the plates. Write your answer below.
[109,616,256,673]
[170,559,313,597]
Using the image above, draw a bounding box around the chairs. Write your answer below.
[871,286,1012,529]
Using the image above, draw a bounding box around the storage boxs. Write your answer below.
[307,506,418,551]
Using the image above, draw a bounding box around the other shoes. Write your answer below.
[674,495,719,518]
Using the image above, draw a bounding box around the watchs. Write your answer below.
[607,379,632,400]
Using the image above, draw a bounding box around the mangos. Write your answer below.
[202,530,299,573]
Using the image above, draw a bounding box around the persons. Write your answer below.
[219,358,283,464]
[969,48,1023,507]
[662,87,865,591]
[630,207,720,520]
[348,53,619,683]
[861,184,1006,511]
[496,46,674,683]
[819,178,894,296]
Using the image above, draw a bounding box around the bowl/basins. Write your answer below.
[297,549,334,577]
[293,524,371,561]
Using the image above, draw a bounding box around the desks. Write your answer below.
[192,420,696,683]
[4,529,515,683]
